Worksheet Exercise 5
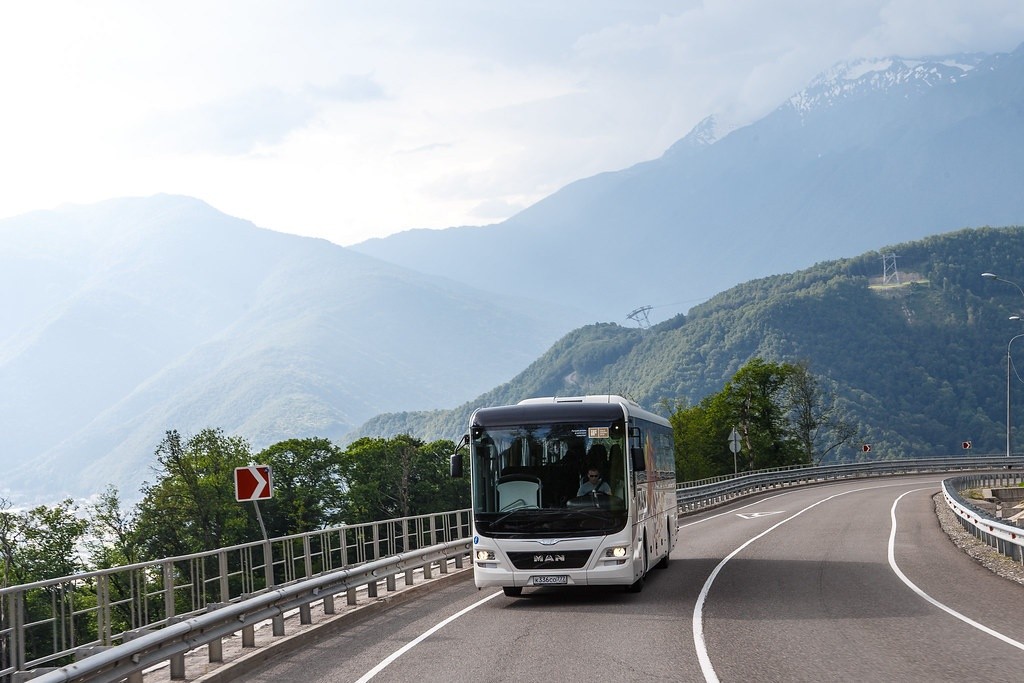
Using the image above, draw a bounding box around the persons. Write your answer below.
[577,468,612,496]
[562,438,586,464]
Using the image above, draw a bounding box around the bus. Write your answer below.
[449,394,680,597]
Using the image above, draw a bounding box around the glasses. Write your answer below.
[590,474,599,478]
